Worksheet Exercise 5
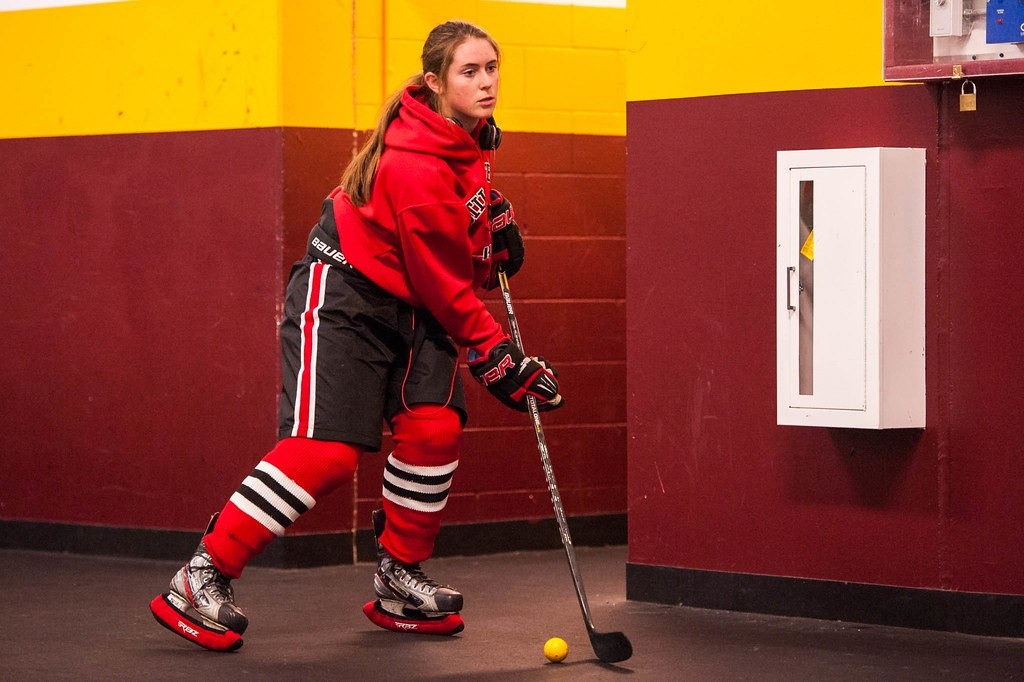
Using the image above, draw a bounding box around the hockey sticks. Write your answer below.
[496,263,634,664]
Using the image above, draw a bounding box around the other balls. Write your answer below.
[544,635,568,663]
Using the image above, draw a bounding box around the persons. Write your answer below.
[149,21,565,651]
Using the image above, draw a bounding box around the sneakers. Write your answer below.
[363,508,463,636]
[149,511,249,652]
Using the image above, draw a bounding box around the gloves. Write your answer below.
[481,188,525,291]
[466,334,564,413]
